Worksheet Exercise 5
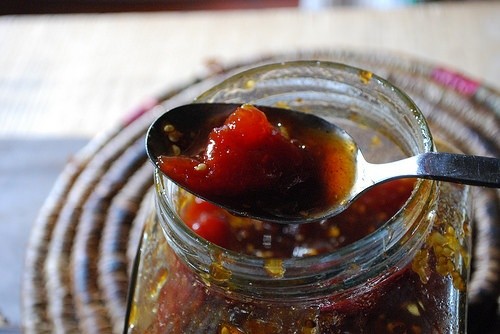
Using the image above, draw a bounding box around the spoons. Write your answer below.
[148,100,500,225]
